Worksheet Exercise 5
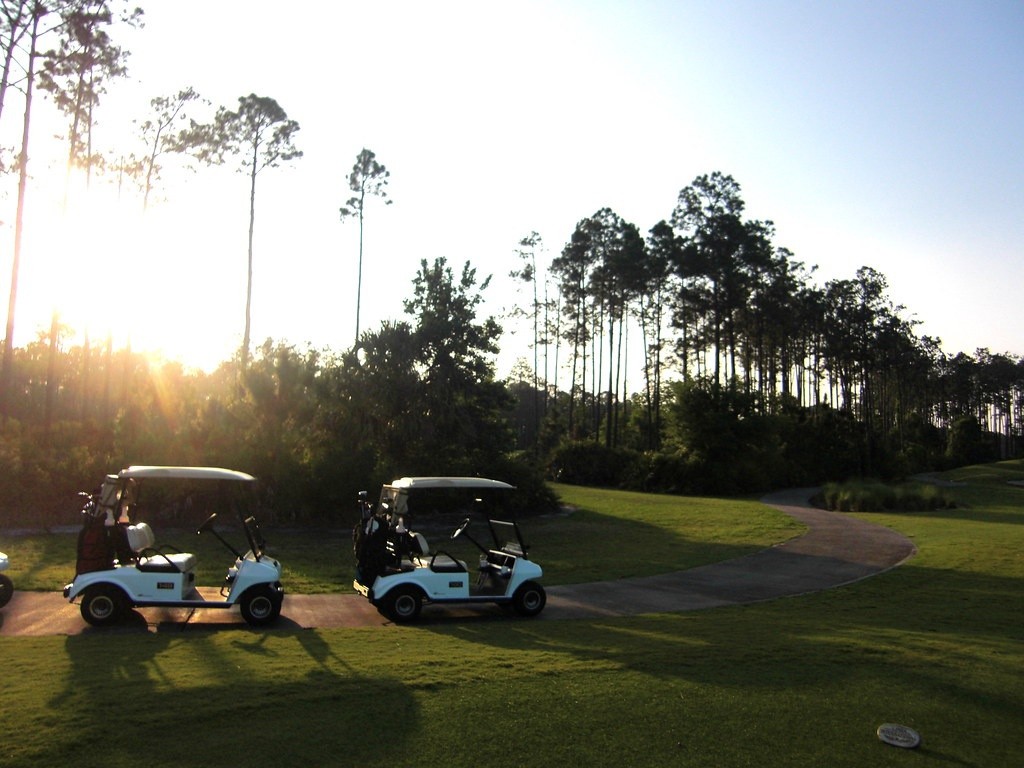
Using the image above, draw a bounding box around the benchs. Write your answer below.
[398,525,469,573]
[127,521,198,573]
